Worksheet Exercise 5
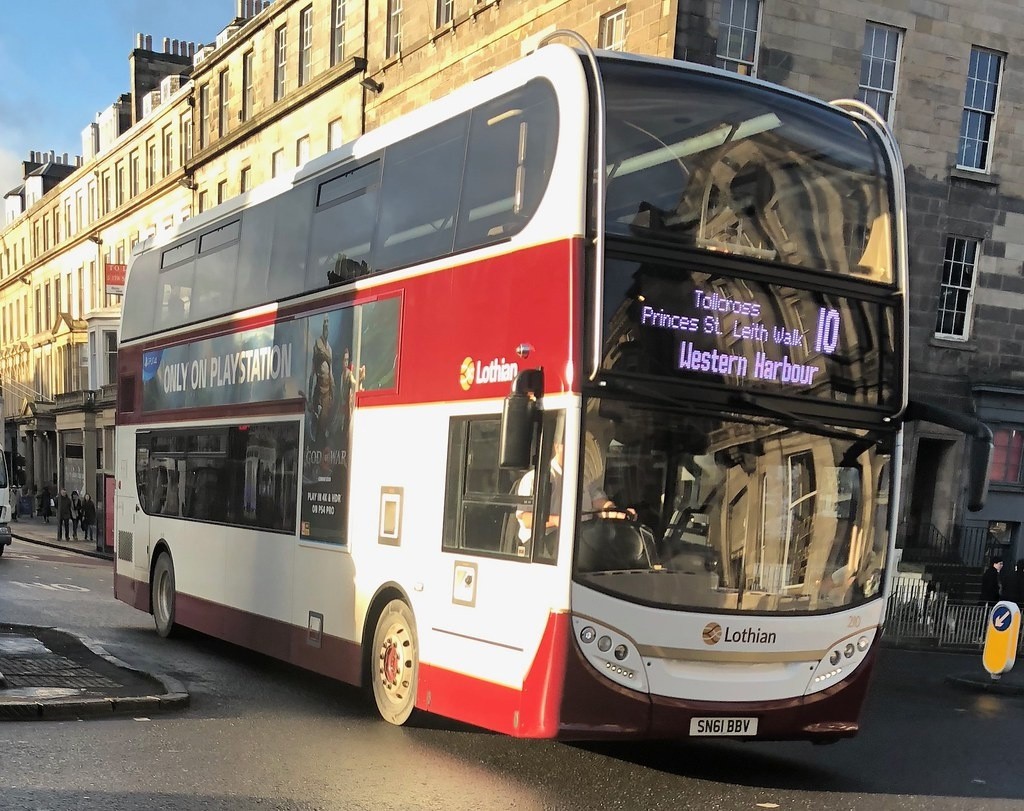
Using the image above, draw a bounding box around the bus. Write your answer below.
[109,28,994,759]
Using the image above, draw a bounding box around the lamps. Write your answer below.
[87,236,103,245]
[18,277,30,285]
[359,77,384,93]
[177,178,198,191]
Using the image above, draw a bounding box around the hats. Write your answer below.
[12,485,16,487]
[989,556,1004,567]
[1014,558,1024,569]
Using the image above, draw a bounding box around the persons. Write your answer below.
[82,492,97,542]
[38,487,52,524]
[69,491,82,542]
[54,488,72,541]
[8,485,23,522]
[307,313,366,483]
[970,556,1004,642]
[516,428,638,557]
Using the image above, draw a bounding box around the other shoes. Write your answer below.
[66,537,70,541]
[85,539,89,541]
[74,538,78,542]
[90,538,93,540]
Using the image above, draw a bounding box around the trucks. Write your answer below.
[0,447,27,561]
